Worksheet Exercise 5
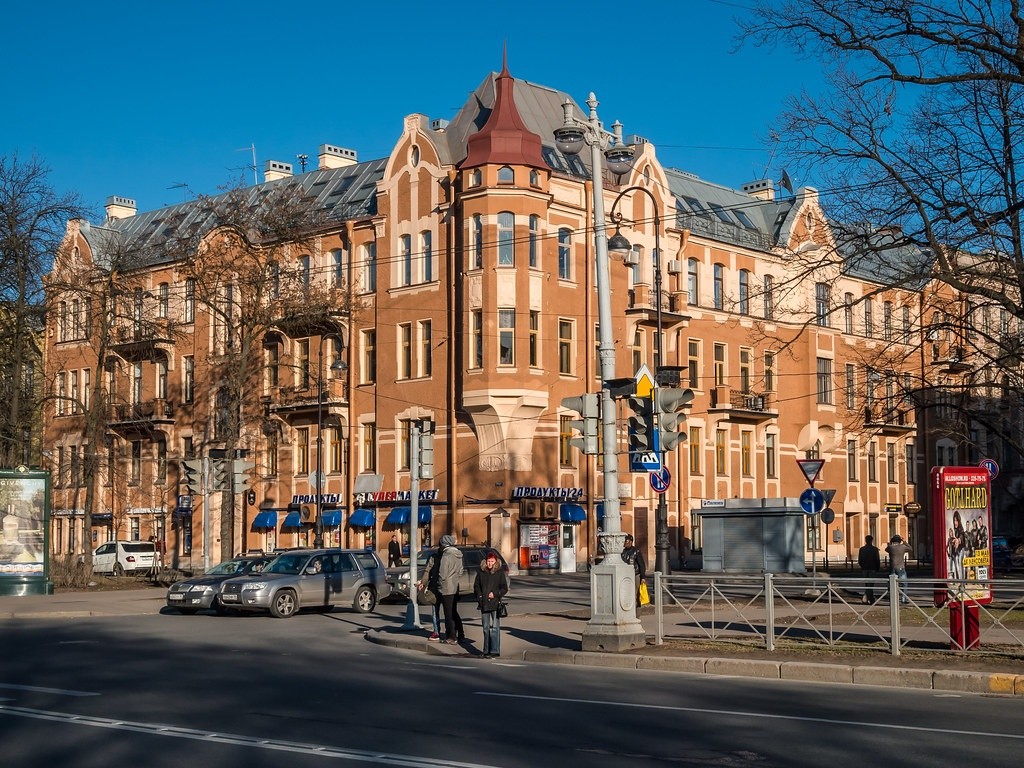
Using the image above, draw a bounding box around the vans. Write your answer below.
[217,546,391,618]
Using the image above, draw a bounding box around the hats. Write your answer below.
[441,535,455,546]
[626,535,633,541]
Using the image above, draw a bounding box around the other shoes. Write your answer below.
[429,633,441,640]
[459,634,465,643]
[442,637,458,645]
[478,651,498,659]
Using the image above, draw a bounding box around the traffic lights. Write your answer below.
[231,460,255,494]
[179,459,201,495]
[214,459,229,491]
[654,386,695,452]
[627,397,655,454]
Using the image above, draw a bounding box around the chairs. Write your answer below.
[323,558,334,574]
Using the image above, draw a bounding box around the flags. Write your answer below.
[781,169,793,195]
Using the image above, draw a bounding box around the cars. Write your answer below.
[167,549,272,615]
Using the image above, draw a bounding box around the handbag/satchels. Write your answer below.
[638,582,650,606]
[418,584,436,605]
[498,600,507,618]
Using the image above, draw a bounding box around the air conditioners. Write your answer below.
[541,502,558,519]
[623,251,640,264]
[519,498,540,519]
[926,330,938,340]
[668,260,680,272]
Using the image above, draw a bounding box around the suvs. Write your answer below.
[992,532,1020,572]
[383,542,511,601]
[76,540,161,577]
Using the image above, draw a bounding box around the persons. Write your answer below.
[474,552,508,659]
[314,561,322,574]
[858,535,881,605]
[418,535,464,644]
[885,535,913,604]
[946,511,988,587]
[156,537,166,554]
[255,562,265,573]
[388,535,401,567]
[621,534,645,617]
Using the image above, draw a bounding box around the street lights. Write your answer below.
[554,97,644,634]
[606,187,678,605]
[313,333,348,549]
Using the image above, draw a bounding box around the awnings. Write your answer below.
[253,511,277,528]
[320,510,341,526]
[386,506,411,524]
[560,504,586,522]
[408,505,431,524]
[285,511,306,527]
[597,504,622,521]
[348,508,376,527]
